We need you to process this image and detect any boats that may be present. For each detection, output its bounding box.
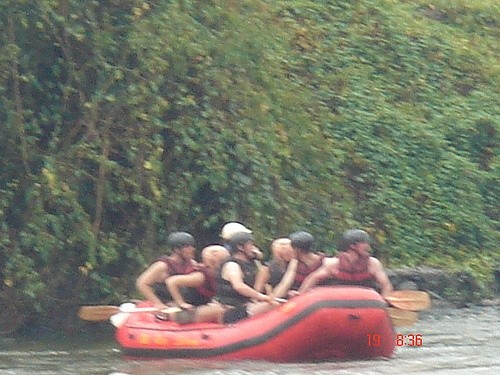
[114,284,397,363]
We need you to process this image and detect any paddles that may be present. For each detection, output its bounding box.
[78,305,182,321]
[272,296,417,326]
[383,289,431,311]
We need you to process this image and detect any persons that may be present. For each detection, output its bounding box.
[138,221,395,325]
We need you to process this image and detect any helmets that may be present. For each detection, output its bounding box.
[229,232,254,252]
[168,231,194,249]
[219,221,254,240]
[343,228,370,244]
[287,229,315,246]
